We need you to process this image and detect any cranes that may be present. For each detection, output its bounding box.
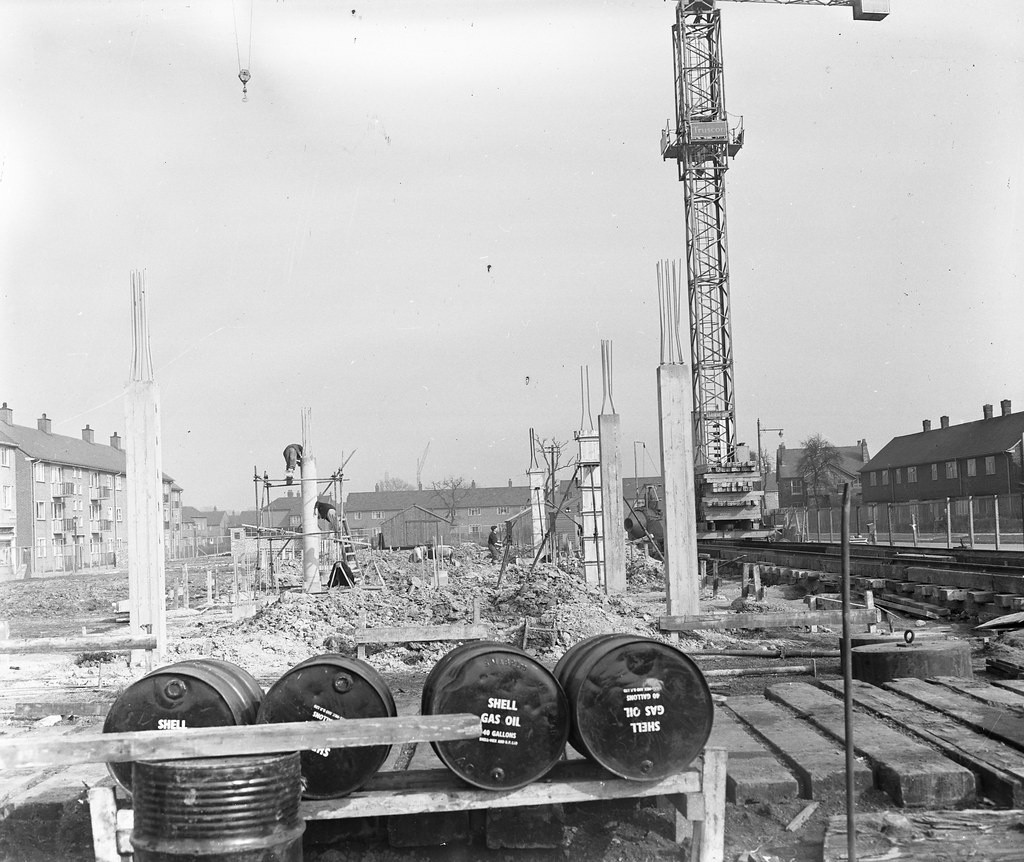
[658,1,889,540]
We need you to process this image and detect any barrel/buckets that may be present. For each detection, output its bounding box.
[421,641,571,791]
[103,658,264,796]
[128,752,306,862]
[553,633,715,783]
[255,654,398,800]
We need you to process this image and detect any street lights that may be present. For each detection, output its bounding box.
[757,418,784,527]
[634,440,646,507]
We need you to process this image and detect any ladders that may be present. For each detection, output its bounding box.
[335,515,363,577]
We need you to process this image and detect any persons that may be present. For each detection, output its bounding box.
[314,501,340,543]
[488,525,500,564]
[283,444,303,484]
